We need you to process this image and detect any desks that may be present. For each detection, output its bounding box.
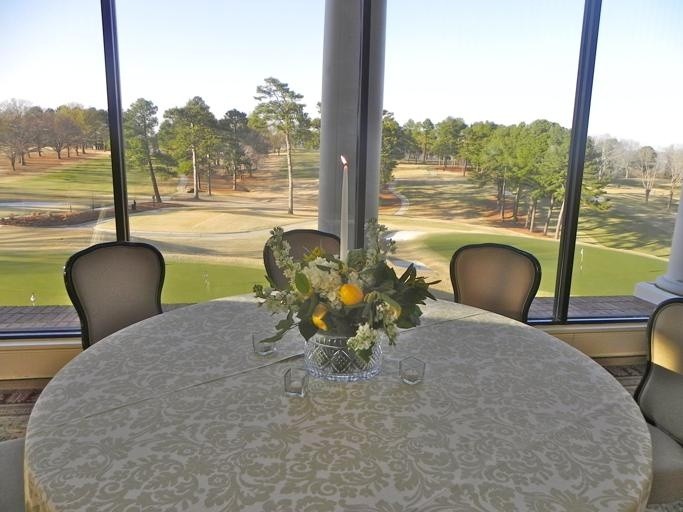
[23,289,655,512]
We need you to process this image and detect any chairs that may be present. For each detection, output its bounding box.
[449,242,542,324]
[62,240,166,352]
[634,296,682,503]
[262,230,340,288]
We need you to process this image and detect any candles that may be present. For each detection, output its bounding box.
[338,154,349,261]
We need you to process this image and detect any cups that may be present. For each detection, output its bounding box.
[253,332,275,354]
[400,359,425,385]
[285,367,309,397]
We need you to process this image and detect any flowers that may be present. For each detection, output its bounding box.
[250,218,442,377]
